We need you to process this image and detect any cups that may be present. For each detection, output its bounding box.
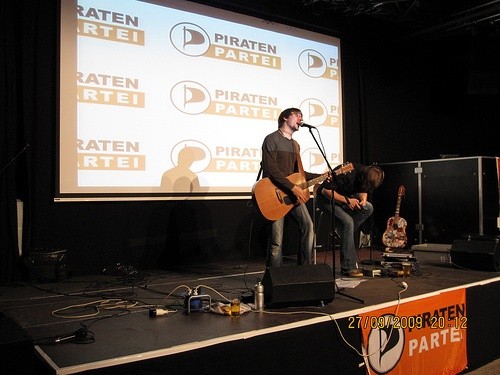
[402,262,411,278]
[231,299,241,319]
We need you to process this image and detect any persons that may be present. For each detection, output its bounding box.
[261,108,332,269]
[316,160,385,277]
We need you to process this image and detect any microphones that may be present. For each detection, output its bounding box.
[54,328,88,343]
[300,122,315,129]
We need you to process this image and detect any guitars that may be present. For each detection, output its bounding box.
[251,162,354,219]
[383,186,409,250]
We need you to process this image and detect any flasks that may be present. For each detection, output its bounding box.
[255,278,265,310]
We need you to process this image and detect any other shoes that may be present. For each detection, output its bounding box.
[330,227,341,240]
[344,270,364,277]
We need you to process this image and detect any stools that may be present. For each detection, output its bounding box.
[315,207,340,264]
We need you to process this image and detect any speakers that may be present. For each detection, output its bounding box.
[260,263,334,310]
[449,239,500,272]
